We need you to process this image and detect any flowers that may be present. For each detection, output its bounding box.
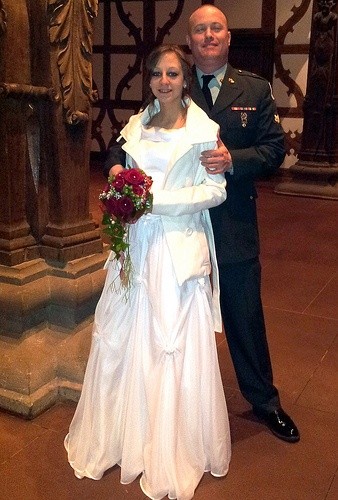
[99,167,155,303]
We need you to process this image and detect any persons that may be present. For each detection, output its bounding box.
[104,4,301,443]
[62,47,232,500]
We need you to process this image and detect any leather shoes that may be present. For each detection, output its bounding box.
[252,406,300,442]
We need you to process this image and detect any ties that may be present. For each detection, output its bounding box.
[201,75,215,111]
